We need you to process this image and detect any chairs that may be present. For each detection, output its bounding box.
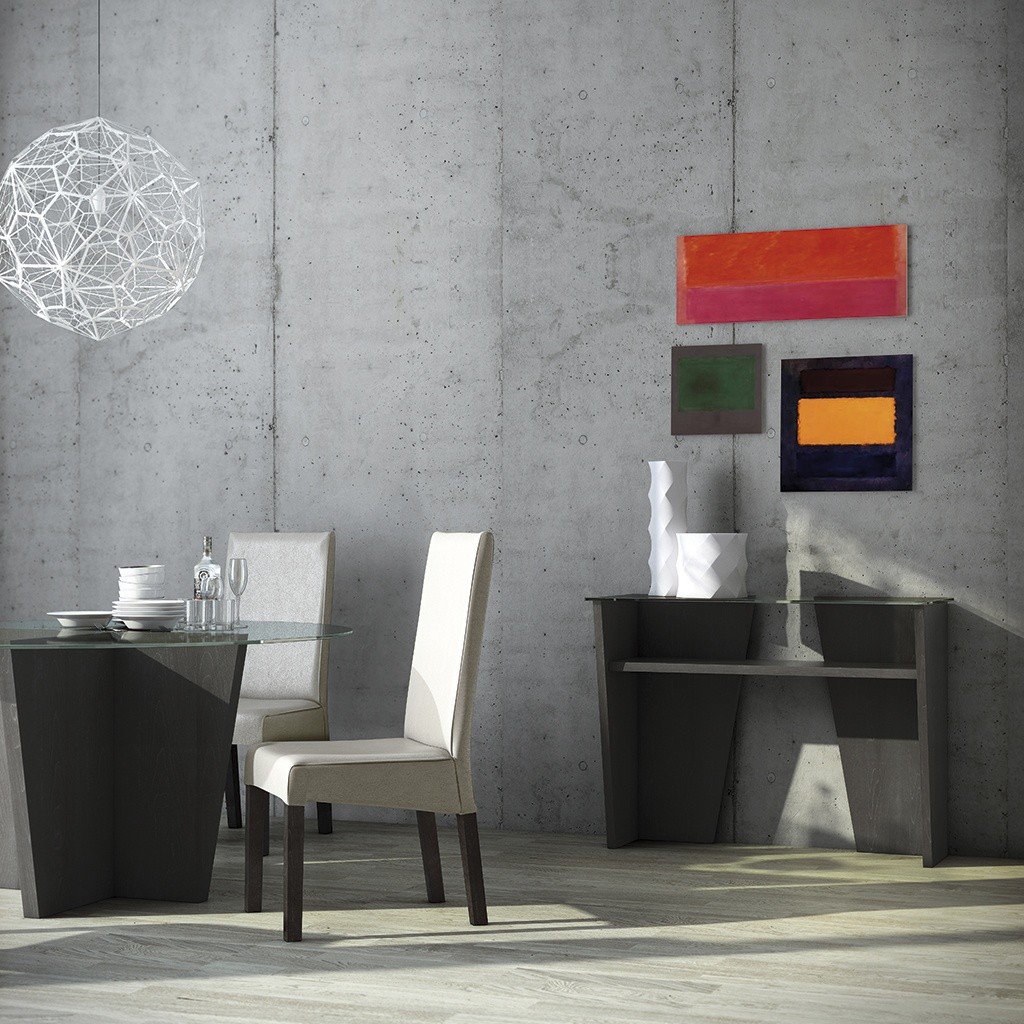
[244,528,496,942]
[216,529,334,834]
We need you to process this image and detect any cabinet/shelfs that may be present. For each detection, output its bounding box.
[587,592,954,869]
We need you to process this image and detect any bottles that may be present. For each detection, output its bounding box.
[192,536,221,600]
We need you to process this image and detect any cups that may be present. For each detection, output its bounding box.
[183,599,236,632]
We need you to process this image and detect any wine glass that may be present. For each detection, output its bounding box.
[228,558,249,628]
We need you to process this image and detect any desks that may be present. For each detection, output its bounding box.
[0,620,351,926]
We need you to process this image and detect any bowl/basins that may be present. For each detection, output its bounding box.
[46,611,112,629]
[112,599,186,632]
[118,564,166,600]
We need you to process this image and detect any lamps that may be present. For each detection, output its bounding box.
[0,0,207,341]
